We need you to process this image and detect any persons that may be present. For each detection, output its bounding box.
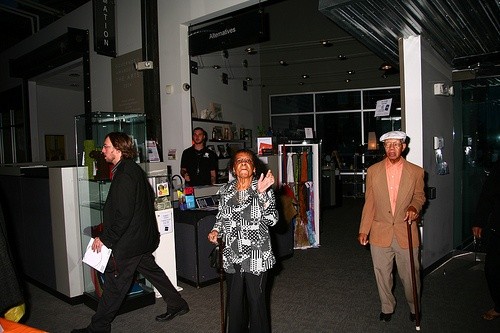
[180,127,219,186]
[71,132,190,333]
[358,131,427,323]
[207,149,280,333]
[471,142,500,322]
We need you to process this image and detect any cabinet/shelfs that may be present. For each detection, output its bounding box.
[192,116,248,184]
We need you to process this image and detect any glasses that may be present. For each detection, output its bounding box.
[102,145,114,149]
[383,142,402,148]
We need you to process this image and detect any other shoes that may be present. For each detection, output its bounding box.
[482,307,499,321]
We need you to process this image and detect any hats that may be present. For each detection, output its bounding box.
[379,131,406,142]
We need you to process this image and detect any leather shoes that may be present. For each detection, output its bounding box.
[379,312,391,322]
[409,311,422,322]
[71,323,94,333]
[156,306,190,321]
